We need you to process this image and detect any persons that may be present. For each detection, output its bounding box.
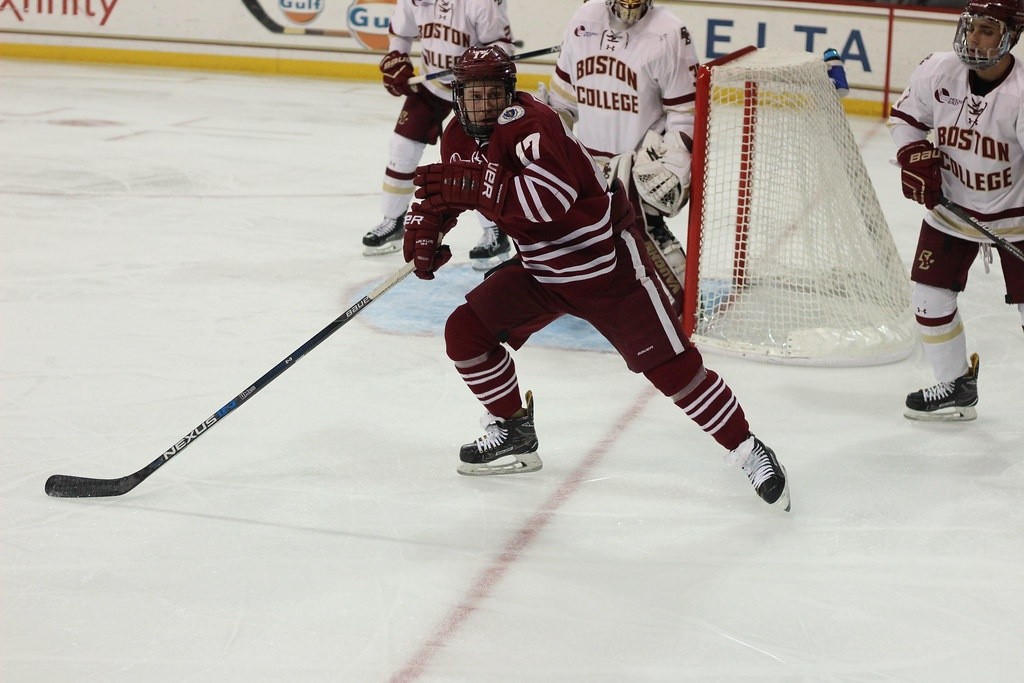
[888,0,1024,421]
[536,0,703,321]
[362,0,513,273]
[403,44,793,514]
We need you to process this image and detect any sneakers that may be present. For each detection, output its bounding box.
[728,431,791,512]
[904,352,980,422]
[469,225,514,270]
[458,390,544,475]
[363,213,408,256]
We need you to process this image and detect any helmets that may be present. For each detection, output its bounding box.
[454,45,517,140]
[965,0,1024,48]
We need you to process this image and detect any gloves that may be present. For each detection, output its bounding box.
[413,158,514,221]
[404,207,457,280]
[896,139,943,211]
[379,48,420,97]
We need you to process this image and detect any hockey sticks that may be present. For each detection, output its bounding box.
[936,194,1024,260]
[642,232,684,320]
[408,44,562,84]
[42,261,414,496]
